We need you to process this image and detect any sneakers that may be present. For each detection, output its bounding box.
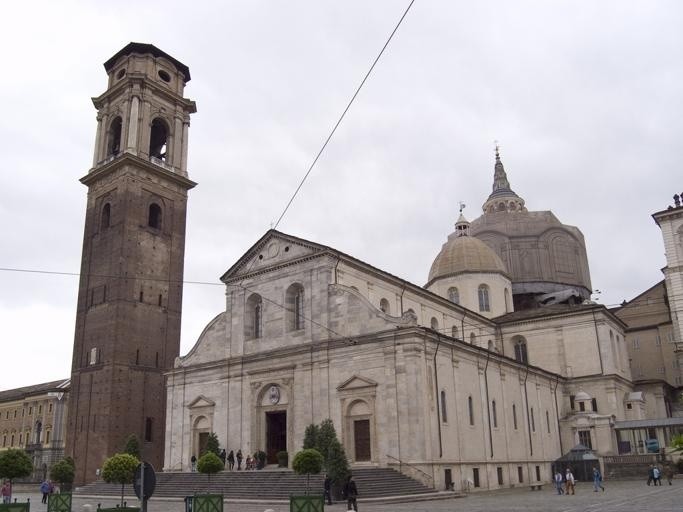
[593,488,604,492]
[565,492,574,495]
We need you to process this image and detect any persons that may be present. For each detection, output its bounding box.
[646,464,656,486]
[564,468,575,495]
[47,480,54,494]
[39,479,50,503]
[346,479,359,511]
[592,466,604,492]
[0,480,11,503]
[653,466,662,486]
[220,448,262,470]
[190,453,197,471]
[665,465,673,485]
[321,473,332,505]
[554,469,564,495]
